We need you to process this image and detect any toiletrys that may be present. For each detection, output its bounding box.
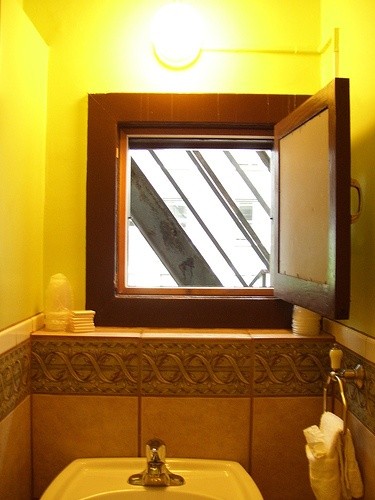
[44,273,74,332]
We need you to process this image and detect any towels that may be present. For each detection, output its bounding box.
[302,411,365,500]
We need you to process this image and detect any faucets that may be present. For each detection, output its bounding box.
[128,438,184,487]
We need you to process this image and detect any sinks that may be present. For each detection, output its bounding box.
[38,457,265,500]
